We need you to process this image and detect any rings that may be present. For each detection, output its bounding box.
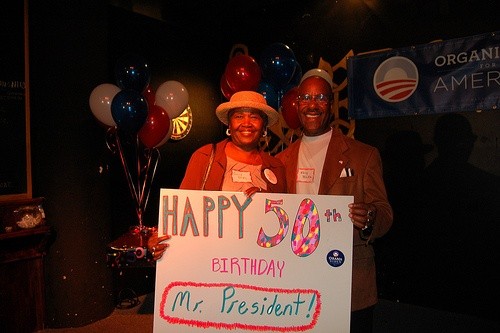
[368,210,373,217]
[152,251,155,256]
[151,246,156,251]
[362,225,368,230]
[367,219,371,224]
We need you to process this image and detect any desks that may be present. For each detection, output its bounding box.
[0,227,49,328]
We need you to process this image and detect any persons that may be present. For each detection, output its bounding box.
[274,75,393,333]
[148,91,285,262]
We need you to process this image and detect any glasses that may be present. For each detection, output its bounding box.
[296,93,330,104]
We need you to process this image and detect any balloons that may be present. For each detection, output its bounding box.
[89,60,189,149]
[220,44,300,111]
[281,87,302,130]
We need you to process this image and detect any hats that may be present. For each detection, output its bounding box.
[216,91,279,127]
